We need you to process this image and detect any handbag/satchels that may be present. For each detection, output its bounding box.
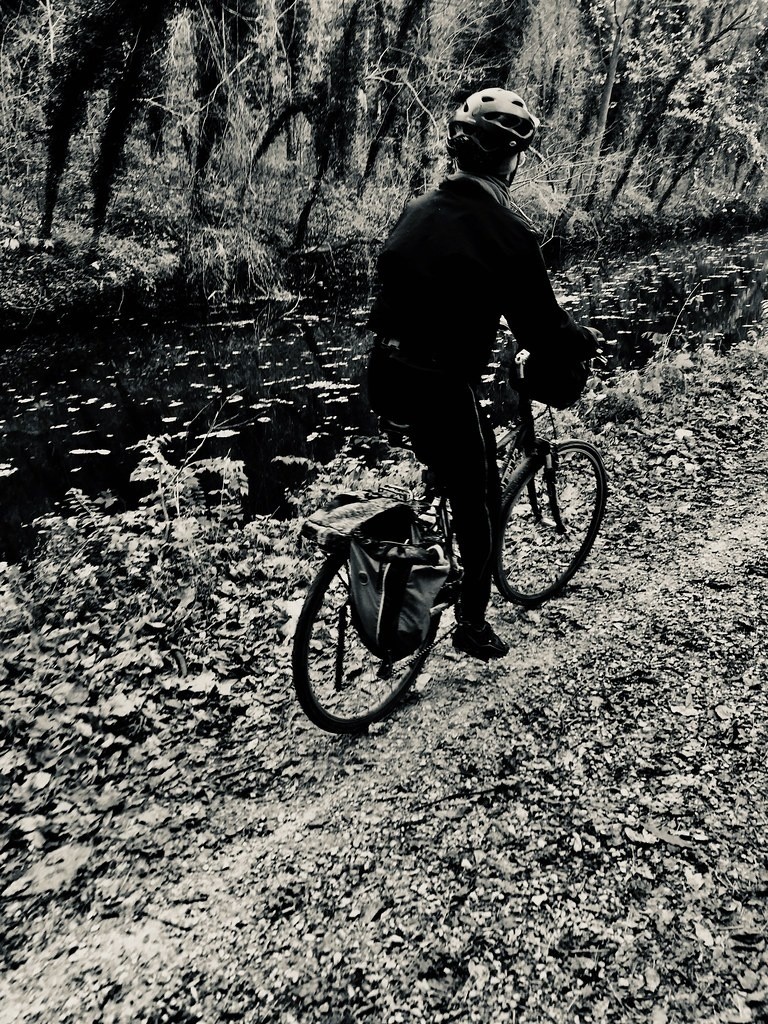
[299,490,449,664]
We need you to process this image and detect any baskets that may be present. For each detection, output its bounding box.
[492,340,590,410]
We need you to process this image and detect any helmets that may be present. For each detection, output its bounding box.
[444,89,534,160]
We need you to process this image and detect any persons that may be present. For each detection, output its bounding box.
[371,87,603,658]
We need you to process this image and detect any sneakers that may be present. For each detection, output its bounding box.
[453,620,511,661]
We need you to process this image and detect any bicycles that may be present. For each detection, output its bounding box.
[290,330,623,733]
